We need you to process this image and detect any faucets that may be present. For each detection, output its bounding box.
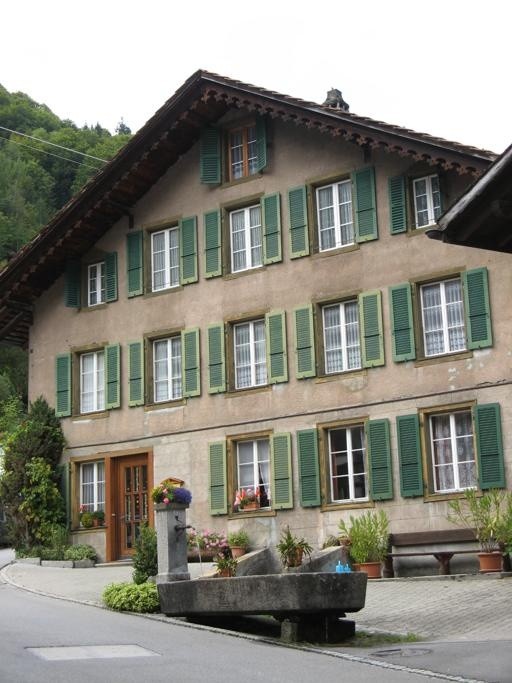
[175,525,196,530]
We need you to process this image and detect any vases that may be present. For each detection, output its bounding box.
[241,501,258,512]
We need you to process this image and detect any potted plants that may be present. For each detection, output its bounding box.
[228,532,249,558]
[79,512,105,528]
[449,486,509,573]
[276,525,310,568]
[213,556,242,577]
[344,508,385,579]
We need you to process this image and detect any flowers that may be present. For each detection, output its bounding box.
[152,484,194,505]
[234,487,258,501]
[186,529,228,548]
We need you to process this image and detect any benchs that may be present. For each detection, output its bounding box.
[386,524,512,578]
